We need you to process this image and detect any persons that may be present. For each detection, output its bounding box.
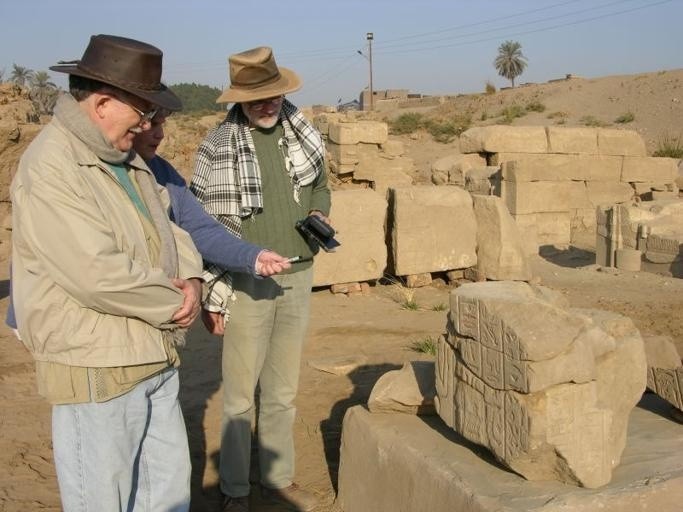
[7,105,292,340]
[9,36,203,512]
[189,46,330,512]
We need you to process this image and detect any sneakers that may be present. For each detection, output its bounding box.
[257,484,319,511]
[221,495,248,512]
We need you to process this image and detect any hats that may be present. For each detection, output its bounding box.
[215,47,301,104]
[49,34,182,110]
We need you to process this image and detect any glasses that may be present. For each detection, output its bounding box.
[246,95,285,111]
[102,91,158,122]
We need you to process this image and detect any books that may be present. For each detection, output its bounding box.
[295,220,341,253]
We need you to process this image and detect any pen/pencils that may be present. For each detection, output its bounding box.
[275,255,302,265]
